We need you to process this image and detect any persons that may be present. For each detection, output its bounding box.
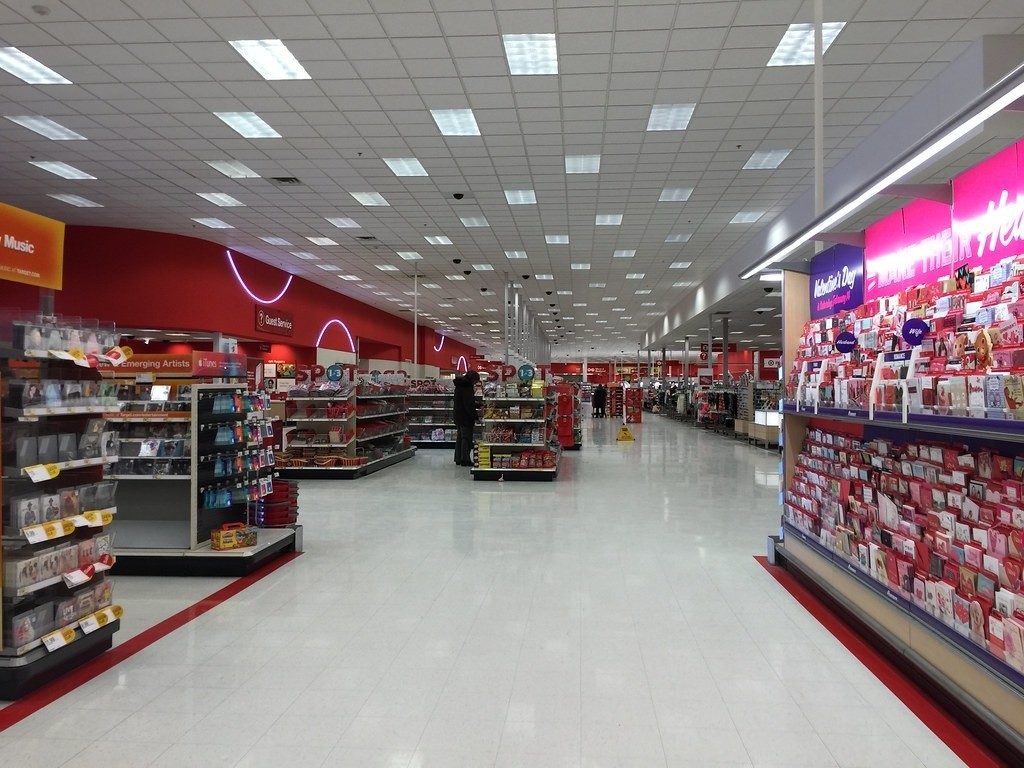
[44,555,58,575]
[671,380,676,390]
[594,384,607,418]
[20,561,37,581]
[106,433,114,456]
[27,384,41,405]
[23,616,35,643]
[24,502,36,526]
[452,370,481,467]
[46,498,57,521]
[938,337,947,356]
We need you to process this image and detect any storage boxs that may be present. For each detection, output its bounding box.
[211,523,259,552]
[473,444,512,469]
[249,476,300,529]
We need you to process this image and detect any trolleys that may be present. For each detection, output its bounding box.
[591,395,607,419]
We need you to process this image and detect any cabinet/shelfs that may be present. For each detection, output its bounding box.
[470,383,562,482]
[542,381,583,451]
[606,388,624,419]
[0,295,121,700]
[738,62,1024,758]
[407,387,458,448]
[107,384,304,576]
[272,384,417,480]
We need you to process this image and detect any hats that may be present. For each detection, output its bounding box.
[48,498,54,502]
[27,502,31,507]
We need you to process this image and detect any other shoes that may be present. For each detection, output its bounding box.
[456,462,460,465]
[460,461,473,466]
